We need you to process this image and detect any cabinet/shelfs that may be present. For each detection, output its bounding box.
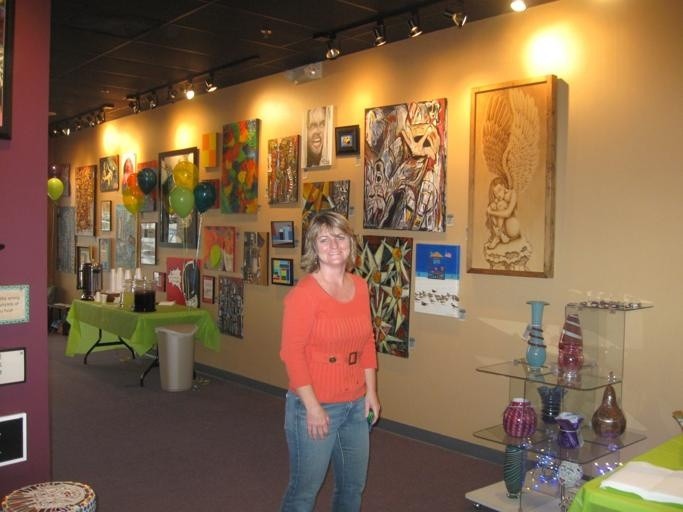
[465,363,646,512]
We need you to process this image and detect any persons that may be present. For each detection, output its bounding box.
[280,212,380,512]
[485,177,521,248]
[306,106,326,167]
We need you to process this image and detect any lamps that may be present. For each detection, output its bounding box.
[49,1,469,137]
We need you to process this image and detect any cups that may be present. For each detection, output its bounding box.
[93,291,101,303]
[100,294,109,304]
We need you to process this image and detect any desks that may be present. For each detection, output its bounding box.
[65,299,221,390]
[570,434,683,512]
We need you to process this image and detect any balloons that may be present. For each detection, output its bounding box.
[47,178,64,201]
[123,161,216,218]
[48,163,64,177]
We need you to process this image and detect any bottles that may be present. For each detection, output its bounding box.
[132,277,159,313]
[120,279,132,313]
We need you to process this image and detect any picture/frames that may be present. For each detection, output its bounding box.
[0,285,30,325]
[270,219,294,248]
[75,245,93,278]
[0,0,14,140]
[201,275,215,304]
[335,126,360,156]
[0,348,27,385]
[271,258,293,286]
[0,412,29,466]
[465,74,558,277]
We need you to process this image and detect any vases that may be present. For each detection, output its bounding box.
[503,301,626,498]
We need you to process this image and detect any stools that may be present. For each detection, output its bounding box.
[0,481,98,512]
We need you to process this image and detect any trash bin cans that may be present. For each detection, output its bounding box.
[154,325,198,393]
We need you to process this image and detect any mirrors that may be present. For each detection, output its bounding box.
[158,147,198,251]
